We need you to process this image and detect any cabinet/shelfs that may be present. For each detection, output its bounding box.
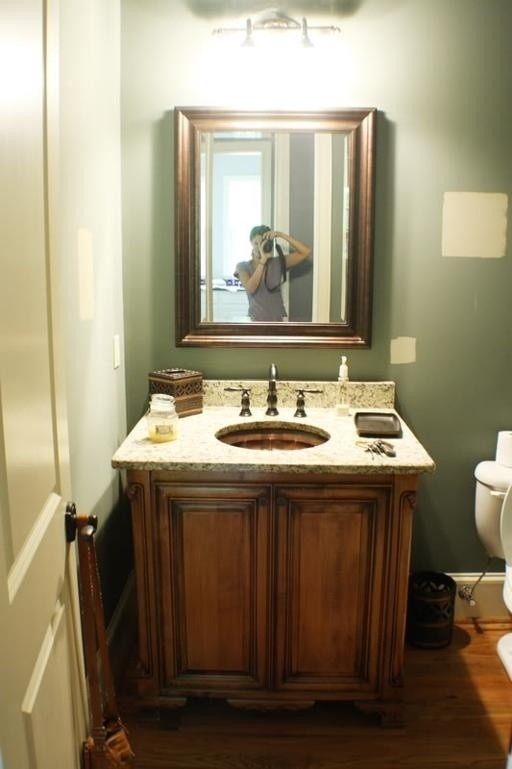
[126,467,396,703]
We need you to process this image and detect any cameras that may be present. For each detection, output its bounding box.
[260,238,273,254]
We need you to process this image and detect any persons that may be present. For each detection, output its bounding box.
[232,226,312,323]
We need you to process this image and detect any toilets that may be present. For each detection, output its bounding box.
[473,459,512,683]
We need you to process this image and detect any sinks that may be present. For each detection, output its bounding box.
[217,421,331,450]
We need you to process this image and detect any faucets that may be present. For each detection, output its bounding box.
[265,364,279,416]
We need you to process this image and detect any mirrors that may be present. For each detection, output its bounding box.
[170,103,378,350]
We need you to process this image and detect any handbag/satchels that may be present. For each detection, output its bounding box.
[83,715,135,769]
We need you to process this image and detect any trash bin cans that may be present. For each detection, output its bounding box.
[408,571,456,648]
